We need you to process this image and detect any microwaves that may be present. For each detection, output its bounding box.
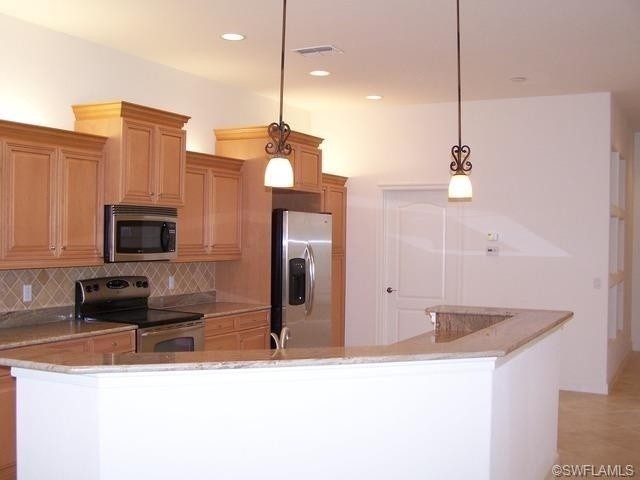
[104,204,178,263]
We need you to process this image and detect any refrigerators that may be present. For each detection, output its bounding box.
[272,209,332,348]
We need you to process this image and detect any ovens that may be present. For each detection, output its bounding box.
[134,319,206,353]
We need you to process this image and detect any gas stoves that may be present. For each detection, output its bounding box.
[81,307,204,327]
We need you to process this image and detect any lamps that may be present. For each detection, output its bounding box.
[447,0,473,203]
[264,2,294,188]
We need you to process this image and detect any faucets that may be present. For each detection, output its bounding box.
[271,349,288,360]
[270,327,292,349]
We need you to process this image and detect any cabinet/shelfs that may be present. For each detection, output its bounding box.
[70,100,193,208]
[208,309,270,351]
[322,173,350,347]
[213,127,326,193]
[0,330,135,480]
[0,120,105,270]
[176,150,251,261]
